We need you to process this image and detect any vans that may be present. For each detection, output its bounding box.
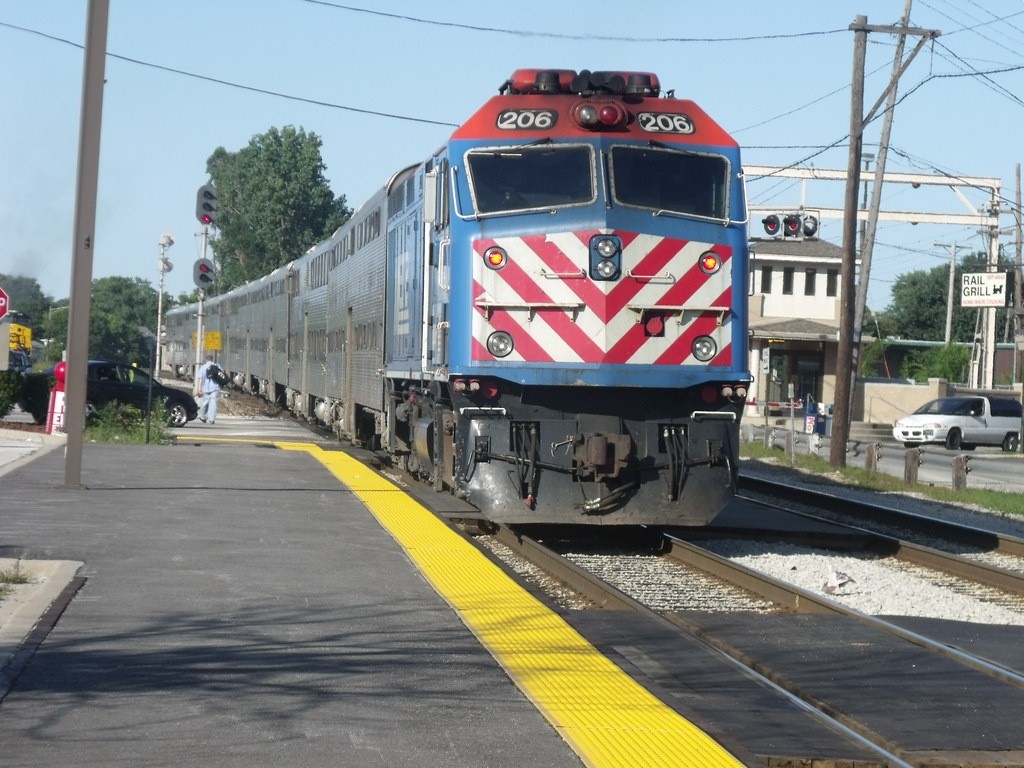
[893,398,1023,452]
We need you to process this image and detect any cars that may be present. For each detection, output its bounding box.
[18,360,198,427]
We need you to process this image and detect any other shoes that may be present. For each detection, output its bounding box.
[198,417,207,423]
[210,420,215,423]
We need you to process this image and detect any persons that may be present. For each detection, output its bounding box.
[198,355,223,423]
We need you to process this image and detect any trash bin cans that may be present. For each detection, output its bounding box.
[826,404,835,437]
[804,402,827,434]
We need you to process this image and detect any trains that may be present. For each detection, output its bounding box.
[165,69,753,525]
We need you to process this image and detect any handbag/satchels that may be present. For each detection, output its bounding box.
[210,364,230,386]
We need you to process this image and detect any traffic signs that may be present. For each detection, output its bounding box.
[762,347,770,374]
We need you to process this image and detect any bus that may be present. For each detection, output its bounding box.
[8,309,32,355]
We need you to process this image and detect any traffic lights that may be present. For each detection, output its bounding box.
[195,185,218,224]
[192,258,214,288]
[762,214,780,235]
[784,215,802,235]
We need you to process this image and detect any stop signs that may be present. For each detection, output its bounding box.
[0,287,10,321]
